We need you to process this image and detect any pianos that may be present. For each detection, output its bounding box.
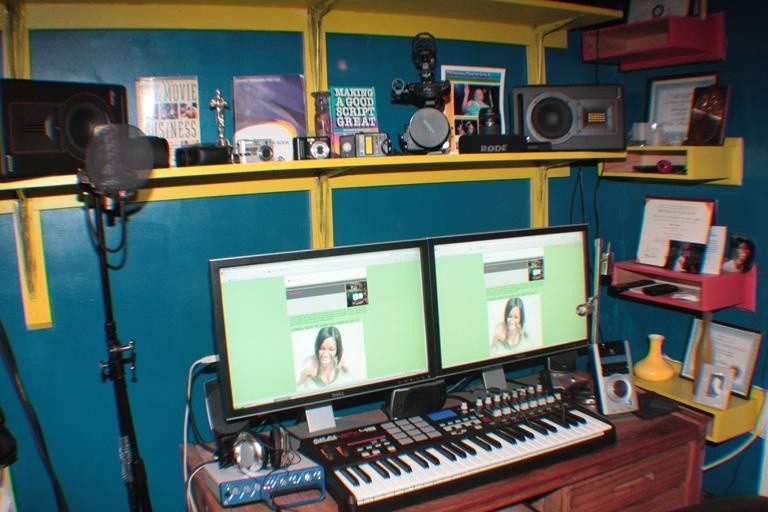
[299,381,618,511]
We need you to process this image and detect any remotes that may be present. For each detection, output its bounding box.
[615,279,656,290]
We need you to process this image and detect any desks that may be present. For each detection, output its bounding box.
[182,369,715,512]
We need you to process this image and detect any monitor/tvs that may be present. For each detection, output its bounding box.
[426,221,593,406]
[209,239,433,448]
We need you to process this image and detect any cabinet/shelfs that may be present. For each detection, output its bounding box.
[597,135,745,186]
[632,349,768,444]
[582,11,728,73]
[608,258,759,313]
[0,0,624,332]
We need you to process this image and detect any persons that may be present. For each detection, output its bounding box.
[461,83,489,117]
[298,327,351,388]
[709,376,722,398]
[720,238,755,274]
[489,297,530,351]
[454,121,475,136]
[672,248,694,273]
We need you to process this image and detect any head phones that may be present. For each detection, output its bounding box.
[212,420,301,476]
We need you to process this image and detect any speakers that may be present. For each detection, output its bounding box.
[0,77,129,182]
[390,379,447,419]
[592,339,640,416]
[509,84,627,151]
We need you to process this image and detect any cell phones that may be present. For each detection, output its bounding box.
[642,284,678,295]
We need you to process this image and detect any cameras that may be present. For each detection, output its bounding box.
[340,136,355,158]
[459,108,527,153]
[293,136,331,160]
[356,133,391,157]
[238,139,276,163]
[391,32,451,154]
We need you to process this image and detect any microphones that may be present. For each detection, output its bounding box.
[70,125,154,267]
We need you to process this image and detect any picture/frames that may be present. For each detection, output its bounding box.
[678,317,763,400]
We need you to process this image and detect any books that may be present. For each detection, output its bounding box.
[232,73,311,161]
[134,75,201,168]
[328,84,379,158]
